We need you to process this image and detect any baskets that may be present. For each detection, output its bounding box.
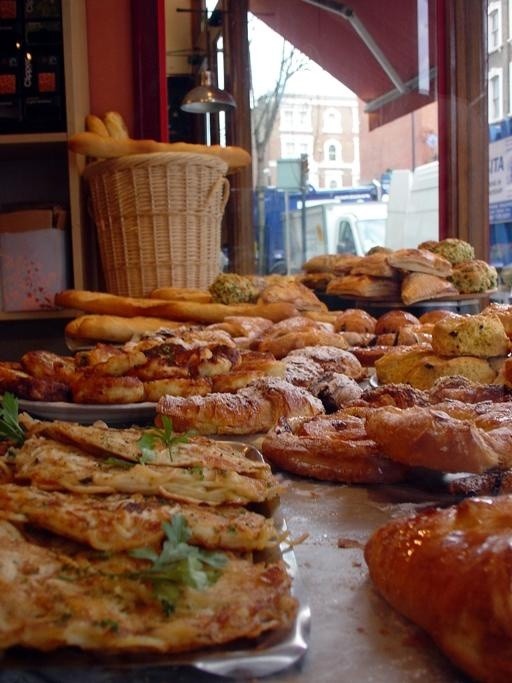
[86,150,230,297]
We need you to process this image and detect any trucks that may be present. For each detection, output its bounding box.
[280,200,386,274]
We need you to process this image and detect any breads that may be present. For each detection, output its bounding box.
[68,111,252,168]
[0,237,512,683]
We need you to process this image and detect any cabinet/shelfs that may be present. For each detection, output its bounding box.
[0,0,92,321]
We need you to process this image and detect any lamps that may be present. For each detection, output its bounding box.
[180,8,237,115]
